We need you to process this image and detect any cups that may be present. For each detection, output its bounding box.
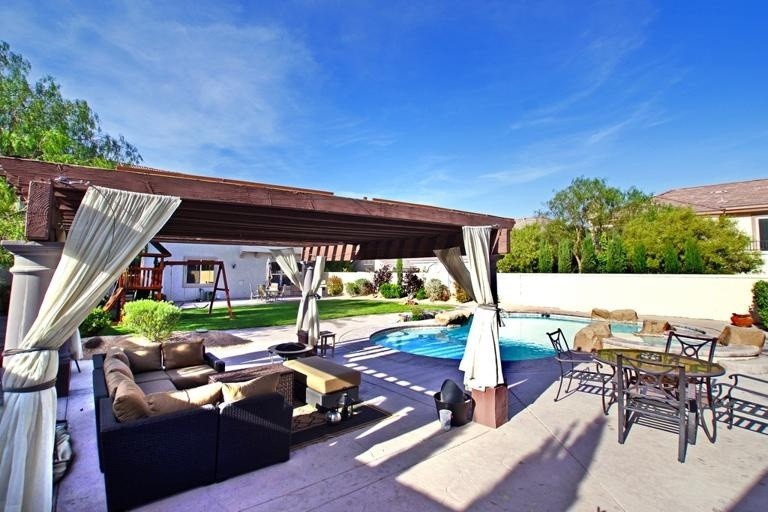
[438,409,453,433]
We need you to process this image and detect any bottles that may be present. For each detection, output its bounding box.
[338,393,354,421]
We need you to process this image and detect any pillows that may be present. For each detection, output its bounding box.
[161,338,205,370]
[220,369,281,403]
[103,357,135,383]
[112,378,148,423]
[104,346,130,368]
[143,381,222,416]
[123,344,161,376]
[104,371,131,398]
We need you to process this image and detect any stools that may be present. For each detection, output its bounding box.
[316,331,336,358]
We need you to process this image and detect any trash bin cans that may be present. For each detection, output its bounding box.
[434,377,476,430]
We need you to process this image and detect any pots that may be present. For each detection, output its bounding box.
[324,408,342,425]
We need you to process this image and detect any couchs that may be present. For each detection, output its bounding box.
[90,345,292,512]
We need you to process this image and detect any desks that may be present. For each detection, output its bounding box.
[587,347,724,407]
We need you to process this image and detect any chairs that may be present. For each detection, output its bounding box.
[710,370,768,447]
[545,327,614,415]
[615,351,695,464]
[664,330,720,412]
[249,282,286,303]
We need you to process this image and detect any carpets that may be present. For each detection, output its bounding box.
[288,397,392,452]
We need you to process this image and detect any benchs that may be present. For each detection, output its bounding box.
[282,356,363,425]
